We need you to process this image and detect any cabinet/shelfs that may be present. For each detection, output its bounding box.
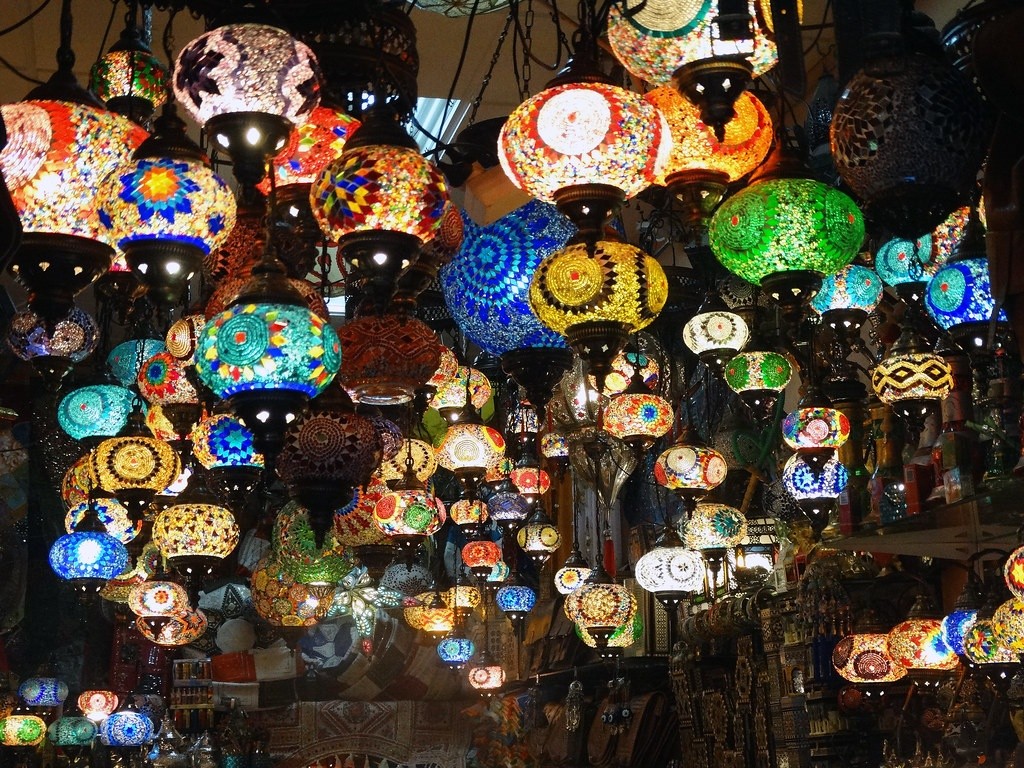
[169,659,214,733]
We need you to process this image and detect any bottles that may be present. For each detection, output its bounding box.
[175,662,210,679]
[170,688,207,704]
[174,709,214,730]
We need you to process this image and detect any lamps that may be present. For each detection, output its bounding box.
[1,0,1021,768]
